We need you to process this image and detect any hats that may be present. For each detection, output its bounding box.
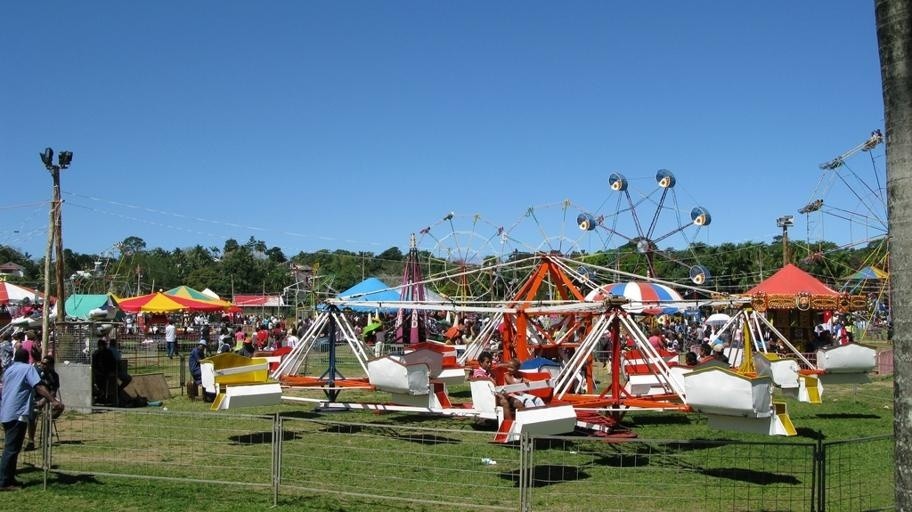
[243,339,252,344]
[197,340,208,347]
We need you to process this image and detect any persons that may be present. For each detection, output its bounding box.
[66,313,165,336]
[0,347,66,493]
[166,312,314,359]
[91,339,131,402]
[0,296,60,419]
[640,308,731,365]
[335,308,498,345]
[474,352,545,420]
[766,293,892,365]
[189,340,206,395]
[528,319,611,394]
[23,354,65,451]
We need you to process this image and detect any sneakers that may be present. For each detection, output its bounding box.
[0,480,24,492]
[23,442,35,451]
